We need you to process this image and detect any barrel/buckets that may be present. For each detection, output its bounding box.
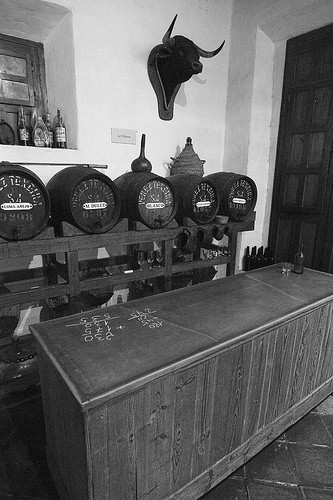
[166,174,220,224]
[0,162,51,241]
[46,167,121,234]
[206,172,257,222]
[113,171,179,230]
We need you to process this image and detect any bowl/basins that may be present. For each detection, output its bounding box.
[215,216,229,224]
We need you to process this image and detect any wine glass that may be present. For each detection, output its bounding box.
[138,252,144,272]
[148,251,155,269]
[156,251,164,268]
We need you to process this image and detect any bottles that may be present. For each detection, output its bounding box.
[243,246,272,271]
[55,109,67,148]
[46,114,53,147]
[30,108,47,146]
[295,243,305,272]
[131,134,152,172]
[18,108,30,146]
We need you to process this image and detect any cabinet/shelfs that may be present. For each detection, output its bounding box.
[0,210,257,415]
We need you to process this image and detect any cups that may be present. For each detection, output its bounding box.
[224,225,233,237]
[195,227,213,245]
[212,226,223,240]
[174,228,195,255]
[200,249,209,261]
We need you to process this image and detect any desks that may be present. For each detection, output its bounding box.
[27,260,333,500]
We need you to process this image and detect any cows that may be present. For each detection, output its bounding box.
[156,13,226,110]
